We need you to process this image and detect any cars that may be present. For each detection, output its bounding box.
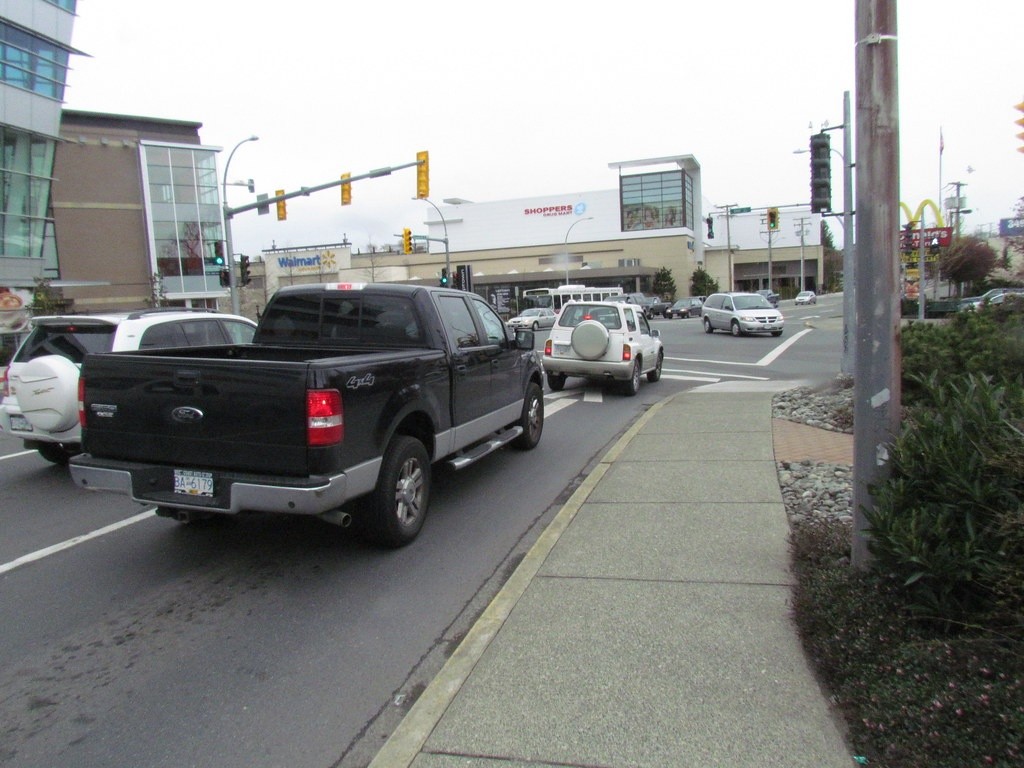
[506,307,560,332]
[755,289,780,308]
[665,297,703,319]
[794,290,816,306]
[900,288,1024,320]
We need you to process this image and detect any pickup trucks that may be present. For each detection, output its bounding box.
[66,282,546,549]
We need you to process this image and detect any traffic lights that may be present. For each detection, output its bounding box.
[403,227,413,255]
[706,217,715,240]
[930,237,940,255]
[900,223,914,256]
[766,208,779,230]
[439,266,449,287]
[218,270,231,287]
[240,254,252,285]
[214,240,225,266]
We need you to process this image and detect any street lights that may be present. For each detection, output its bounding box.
[565,216,594,285]
[412,196,451,287]
[220,136,261,343]
[946,208,973,298]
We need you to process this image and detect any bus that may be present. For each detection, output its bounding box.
[522,285,623,325]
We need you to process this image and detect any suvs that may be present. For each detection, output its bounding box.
[588,291,674,324]
[541,299,665,397]
[0,304,259,464]
[701,291,785,337]
